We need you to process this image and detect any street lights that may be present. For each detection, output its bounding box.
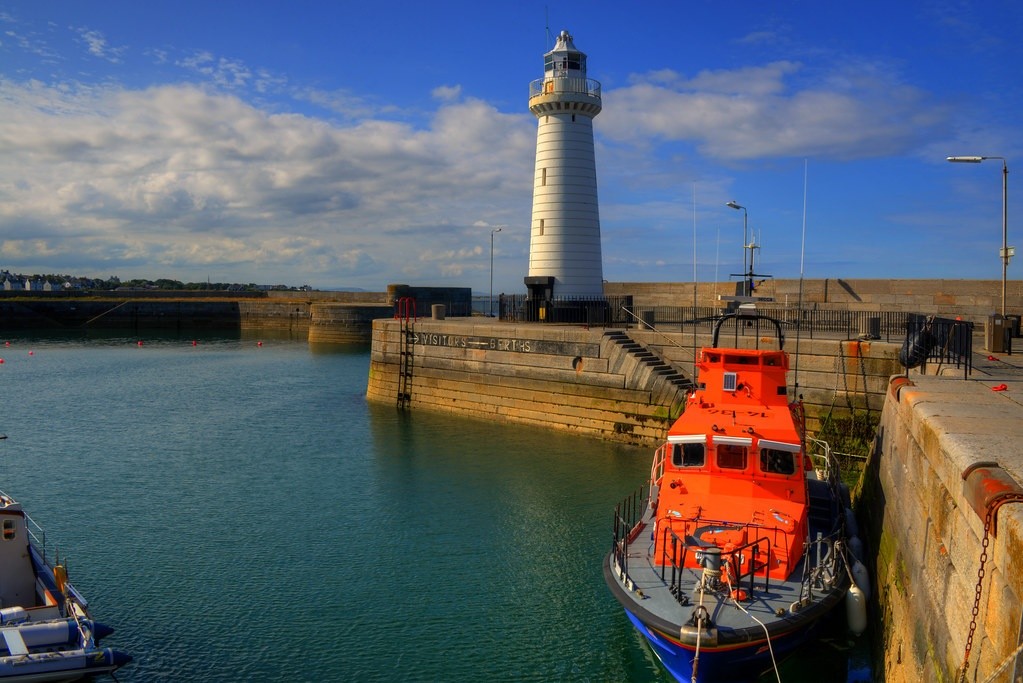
[487,227,501,318]
[726,201,747,296]
[947,154,1008,320]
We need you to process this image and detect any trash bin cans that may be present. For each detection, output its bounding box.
[984,313,1004,352]
[1006,314,1021,338]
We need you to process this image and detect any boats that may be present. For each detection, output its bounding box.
[603,315,869,682]
[0,481,116,683]
